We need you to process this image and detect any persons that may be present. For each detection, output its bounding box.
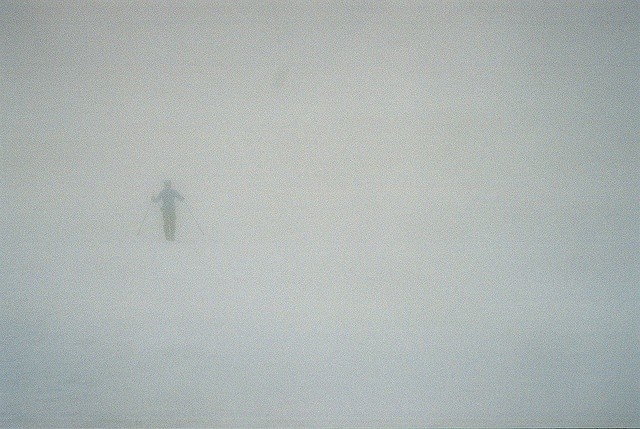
[151,178,184,242]
